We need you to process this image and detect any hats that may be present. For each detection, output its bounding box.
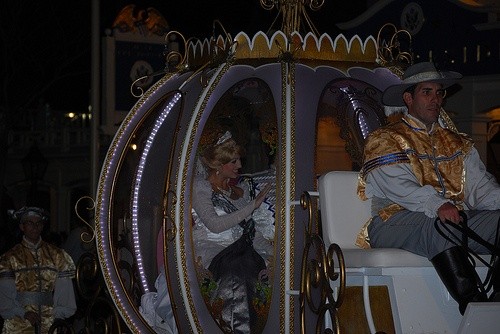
[14,206,48,222]
[382,62,463,107]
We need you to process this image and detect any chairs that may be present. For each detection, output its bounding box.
[300,168,500,334]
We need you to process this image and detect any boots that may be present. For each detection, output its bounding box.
[431,247,489,317]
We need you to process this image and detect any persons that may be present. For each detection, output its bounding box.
[357,62,500,318]
[0,205,78,334]
[139,122,276,334]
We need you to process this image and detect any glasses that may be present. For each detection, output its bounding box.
[22,220,42,225]
